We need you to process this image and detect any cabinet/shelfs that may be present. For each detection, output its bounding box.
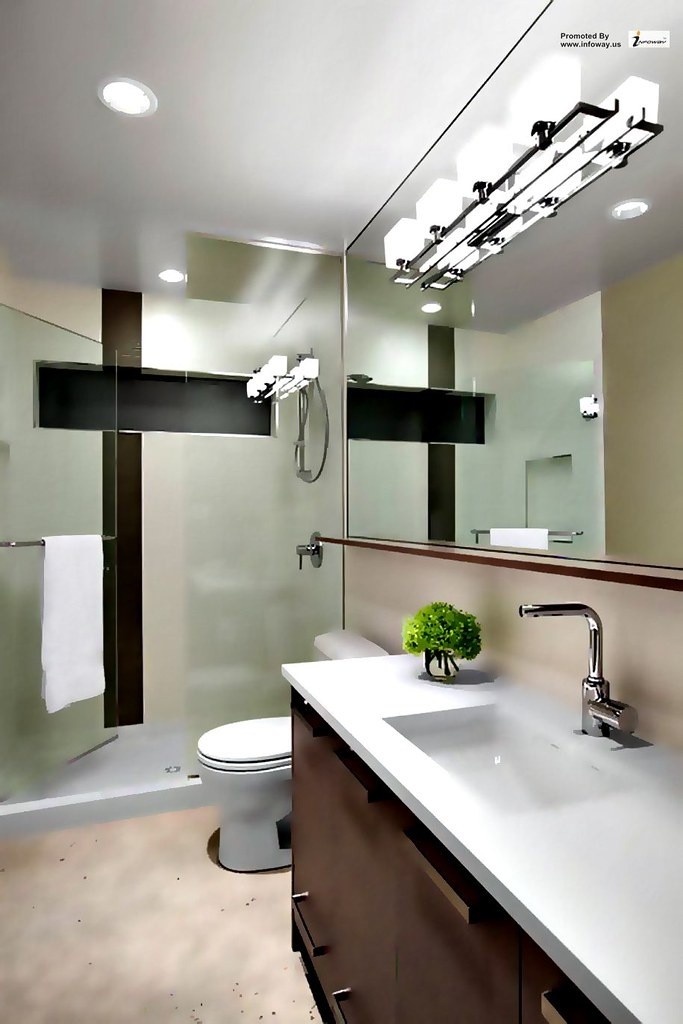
[291,685,610,1024]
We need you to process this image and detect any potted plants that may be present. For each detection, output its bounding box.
[401,602,483,682]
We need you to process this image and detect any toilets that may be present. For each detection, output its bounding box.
[196,628,390,873]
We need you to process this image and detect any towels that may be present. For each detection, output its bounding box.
[489,528,549,551]
[40,534,105,714]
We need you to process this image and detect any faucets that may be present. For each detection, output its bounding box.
[518,602,640,739]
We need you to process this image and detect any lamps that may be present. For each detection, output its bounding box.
[383,54,664,293]
[246,354,319,403]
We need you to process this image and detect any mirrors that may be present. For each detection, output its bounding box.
[346,0,683,570]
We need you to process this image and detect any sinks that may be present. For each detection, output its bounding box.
[380,702,646,818]
